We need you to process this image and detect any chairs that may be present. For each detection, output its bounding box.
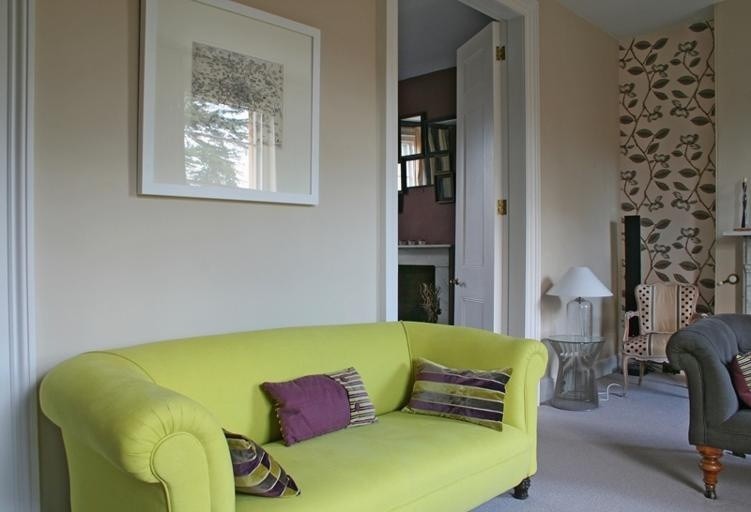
[621,280,709,398]
[664,312,751,500]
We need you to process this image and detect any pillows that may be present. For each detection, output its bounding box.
[726,349,751,410]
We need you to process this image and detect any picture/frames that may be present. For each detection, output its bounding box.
[137,0,322,210]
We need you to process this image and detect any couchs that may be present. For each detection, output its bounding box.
[35,319,552,512]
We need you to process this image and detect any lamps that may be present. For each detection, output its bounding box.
[544,265,614,338]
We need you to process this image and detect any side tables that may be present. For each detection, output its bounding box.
[545,334,605,412]
